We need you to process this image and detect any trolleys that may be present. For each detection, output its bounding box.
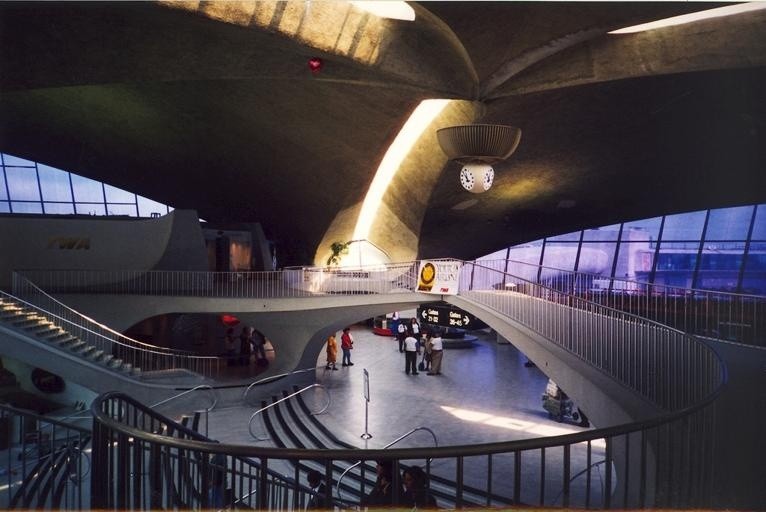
[548,391,579,422]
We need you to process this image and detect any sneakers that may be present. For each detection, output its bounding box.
[326,366,332,369]
[342,362,354,366]
[333,367,338,370]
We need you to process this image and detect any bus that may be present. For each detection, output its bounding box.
[635,248,766,289]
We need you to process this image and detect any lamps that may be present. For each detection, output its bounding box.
[433,100,523,194]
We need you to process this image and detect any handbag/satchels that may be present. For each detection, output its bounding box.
[418,361,424,371]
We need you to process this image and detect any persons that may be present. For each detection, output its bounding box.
[364,458,404,511]
[206,439,226,510]
[340,328,354,366]
[397,317,443,376]
[305,470,327,512]
[326,332,339,370]
[250,330,267,364]
[396,465,438,512]
[225,328,240,367]
[239,326,252,367]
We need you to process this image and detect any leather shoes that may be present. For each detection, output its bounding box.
[427,372,441,375]
